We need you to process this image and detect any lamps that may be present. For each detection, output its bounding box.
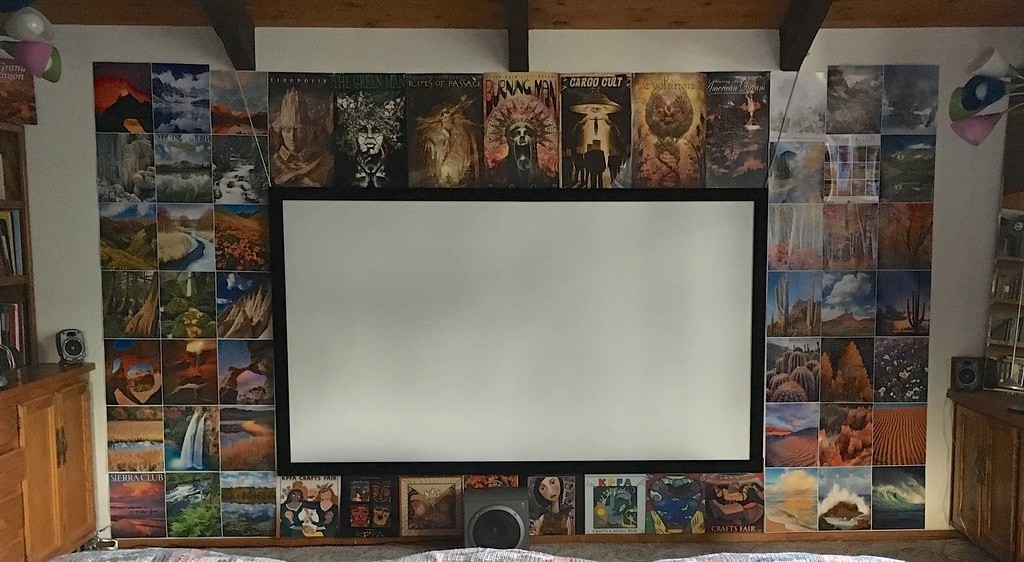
[1,6,64,85]
[950,45,1023,147]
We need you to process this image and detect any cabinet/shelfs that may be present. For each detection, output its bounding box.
[1,123,39,373]
[2,360,99,562]
[947,385,1024,562]
[979,68,1023,395]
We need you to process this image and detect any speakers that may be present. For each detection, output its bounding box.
[55,329,86,367]
[462,488,530,550]
[951,356,998,391]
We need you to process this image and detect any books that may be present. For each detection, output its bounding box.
[989,308,1024,344]
[0,146,24,202]
[986,350,1024,391]
[1,208,28,279]
[0,301,29,369]
[994,263,1024,304]
[998,219,1023,259]
